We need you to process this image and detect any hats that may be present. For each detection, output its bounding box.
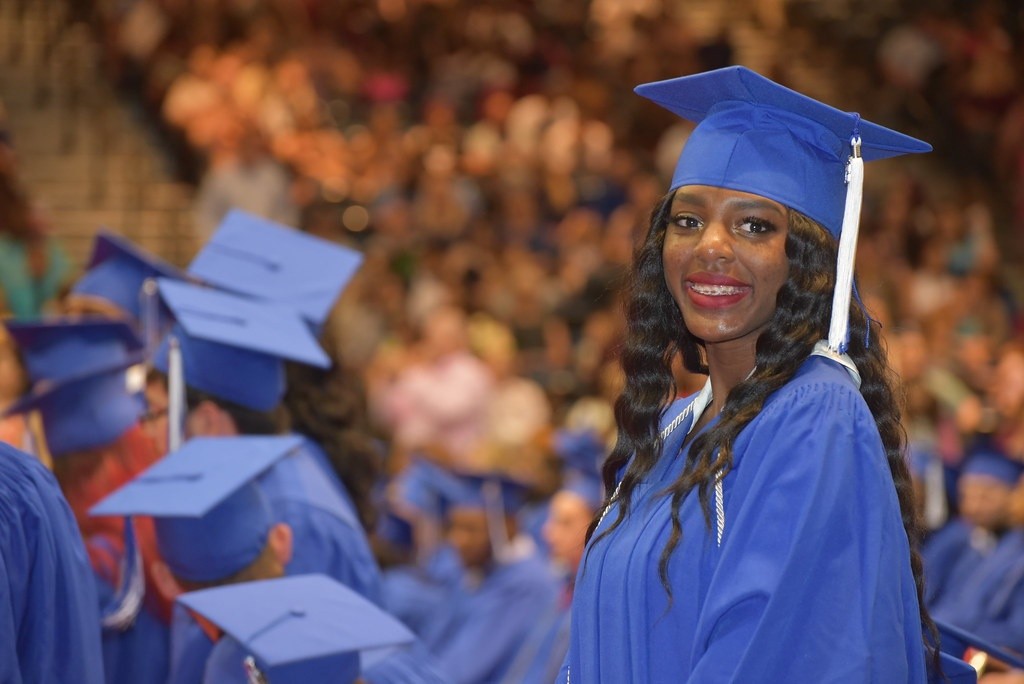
[2,315,147,456]
[634,64,933,356]
[88,433,416,684]
[958,444,1020,484]
[71,208,365,418]
[368,428,630,550]
[925,612,1024,684]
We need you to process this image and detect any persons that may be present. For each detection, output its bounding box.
[898,430,1024,684]
[364,431,614,684]
[548,64,924,684]
[0,0,1024,484]
[0,206,426,684]
